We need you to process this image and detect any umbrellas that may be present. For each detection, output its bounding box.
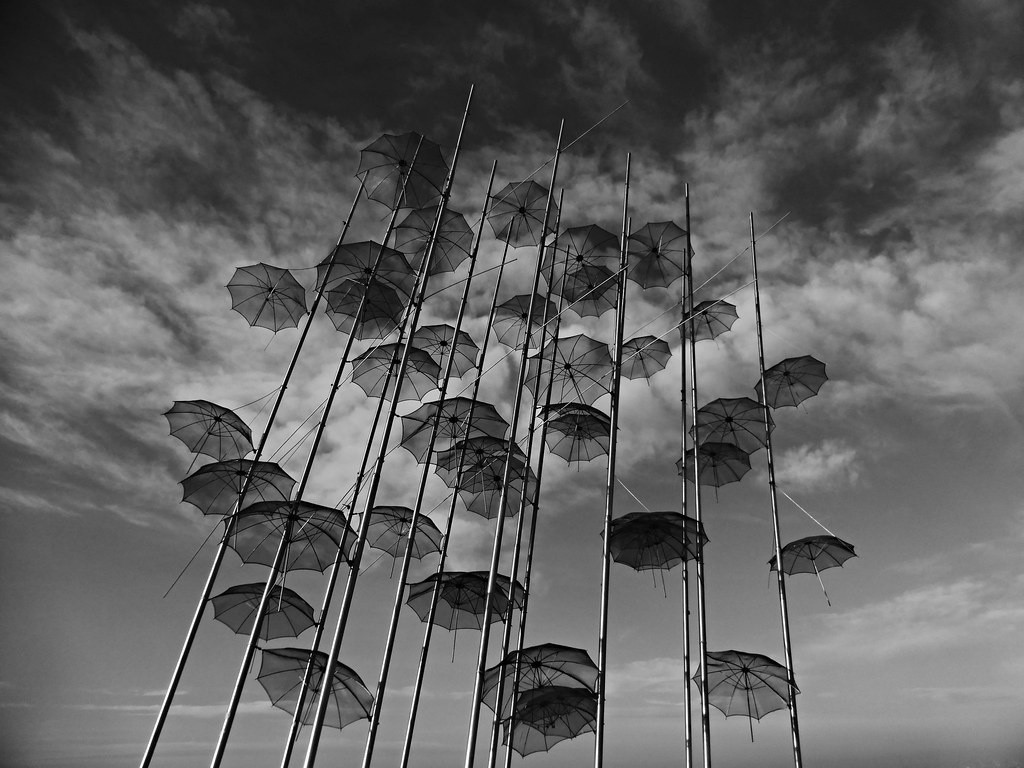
[258,648,374,743]
[690,649,801,742]
[477,642,599,722]
[766,534,860,608]
[754,352,829,415]
[497,683,598,758]
[155,131,777,641]
